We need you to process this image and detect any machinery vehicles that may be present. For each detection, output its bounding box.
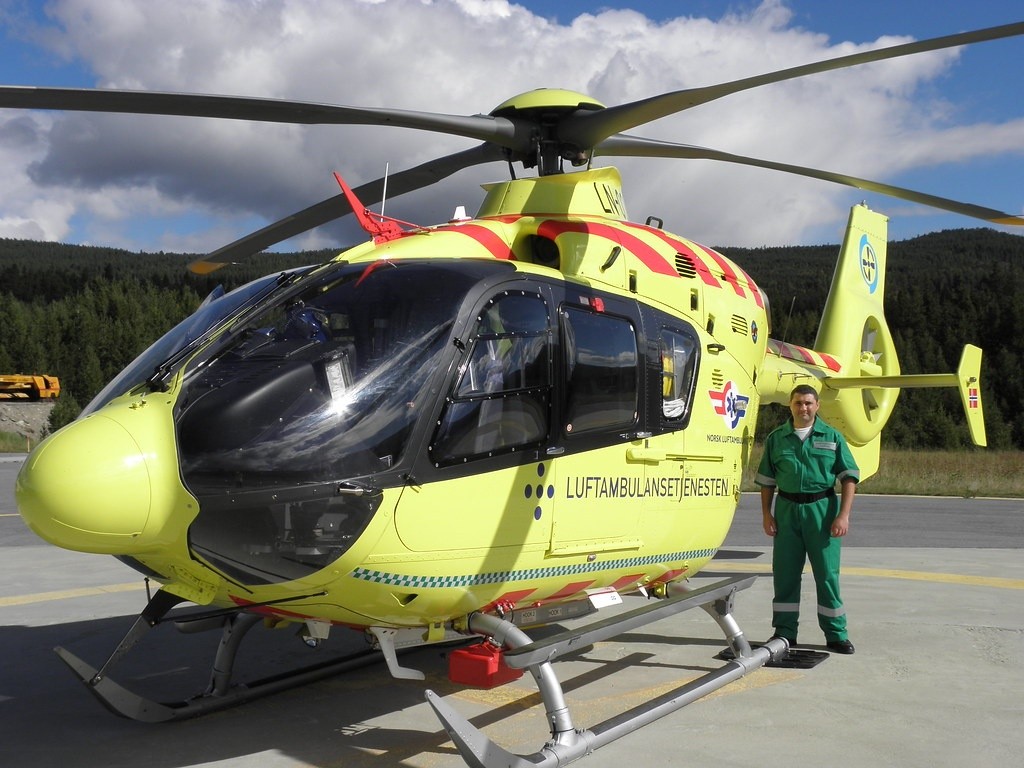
[1,374,60,402]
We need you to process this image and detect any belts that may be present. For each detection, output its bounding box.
[779,488,834,503]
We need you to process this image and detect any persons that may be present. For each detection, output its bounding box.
[754,385,860,654]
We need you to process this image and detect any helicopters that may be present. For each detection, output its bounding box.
[1,21,1020,768]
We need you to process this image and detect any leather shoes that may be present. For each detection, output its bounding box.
[768,635,798,646]
[826,640,855,654]
